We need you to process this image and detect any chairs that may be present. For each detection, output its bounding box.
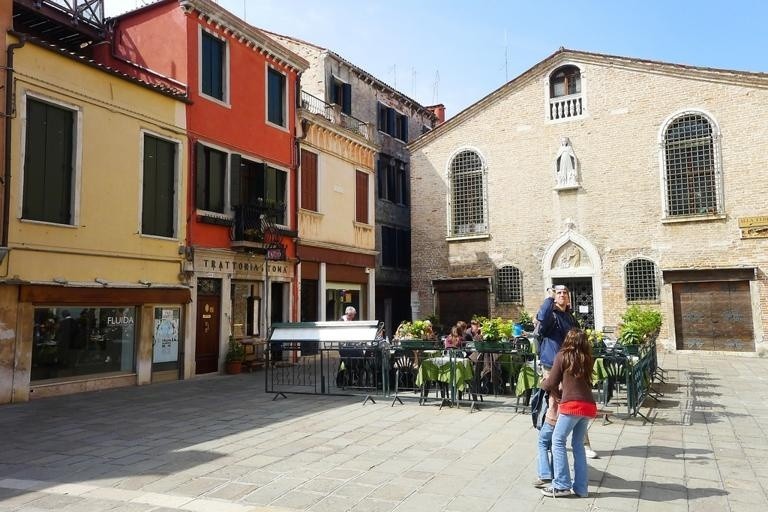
[337,332,647,409]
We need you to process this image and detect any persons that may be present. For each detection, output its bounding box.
[554,138,579,186]
[34,309,124,372]
[535,285,597,459]
[570,244,581,267]
[540,329,597,497]
[337,307,357,354]
[533,375,561,489]
[560,250,569,265]
[375,317,482,361]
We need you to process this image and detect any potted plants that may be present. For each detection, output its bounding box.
[397,321,439,348]
[471,313,515,351]
[616,321,641,354]
[224,334,244,374]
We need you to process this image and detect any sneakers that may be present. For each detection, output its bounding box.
[584,445,597,458]
[532,479,588,498]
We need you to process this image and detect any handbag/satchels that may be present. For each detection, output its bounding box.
[530,389,550,430]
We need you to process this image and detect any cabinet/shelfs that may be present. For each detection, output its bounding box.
[241,339,268,371]
